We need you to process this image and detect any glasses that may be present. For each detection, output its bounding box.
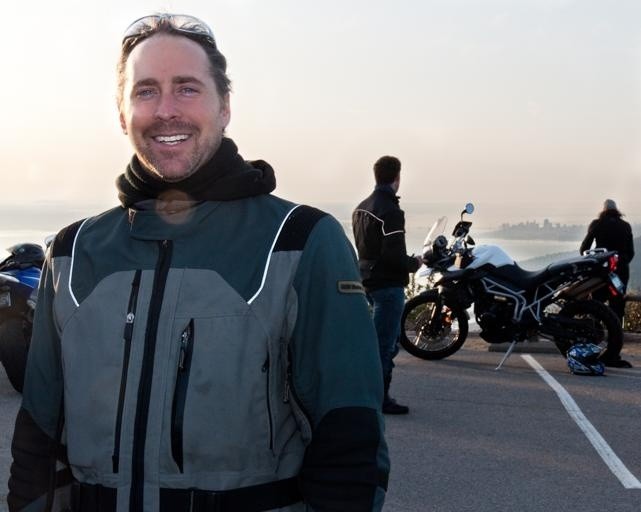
[120,13,217,51]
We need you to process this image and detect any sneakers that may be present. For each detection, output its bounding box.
[606,356,633,367]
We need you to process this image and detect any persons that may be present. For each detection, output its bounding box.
[352,155,423,414]
[579,199,635,367]
[6,14,390,512]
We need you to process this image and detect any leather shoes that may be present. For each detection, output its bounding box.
[387,405,410,416]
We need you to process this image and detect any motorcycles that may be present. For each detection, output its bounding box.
[397,203,624,366]
[0,233,55,395]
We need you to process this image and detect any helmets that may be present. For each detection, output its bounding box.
[566,343,608,375]
